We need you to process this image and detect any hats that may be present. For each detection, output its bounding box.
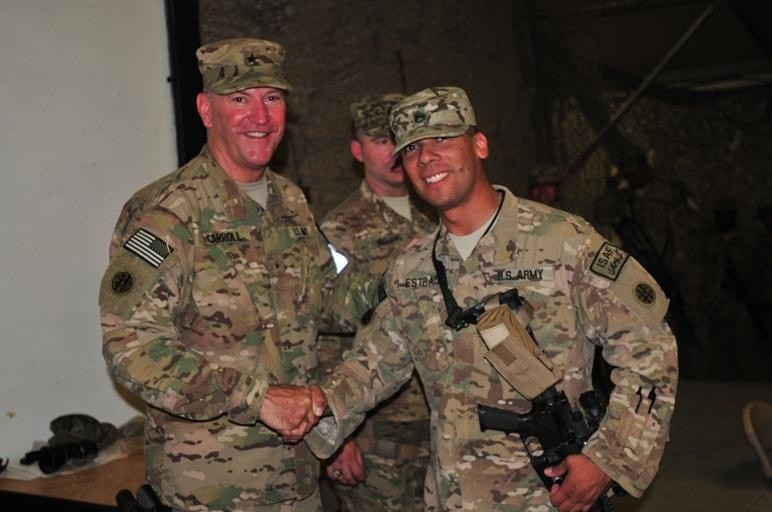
[528,166,559,188]
[390,87,475,159]
[348,93,406,139]
[196,37,292,96]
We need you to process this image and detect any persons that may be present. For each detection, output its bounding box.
[273,85,679,512]
[98,36,390,512]
[522,163,560,206]
[306,93,434,512]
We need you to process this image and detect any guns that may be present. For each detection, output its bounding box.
[478,386,625,510]
[594,216,696,361]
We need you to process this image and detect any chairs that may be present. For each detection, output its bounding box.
[742,400,771,480]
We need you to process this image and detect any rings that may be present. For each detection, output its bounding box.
[331,470,343,478]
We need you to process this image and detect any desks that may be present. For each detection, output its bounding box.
[0,432,144,511]
